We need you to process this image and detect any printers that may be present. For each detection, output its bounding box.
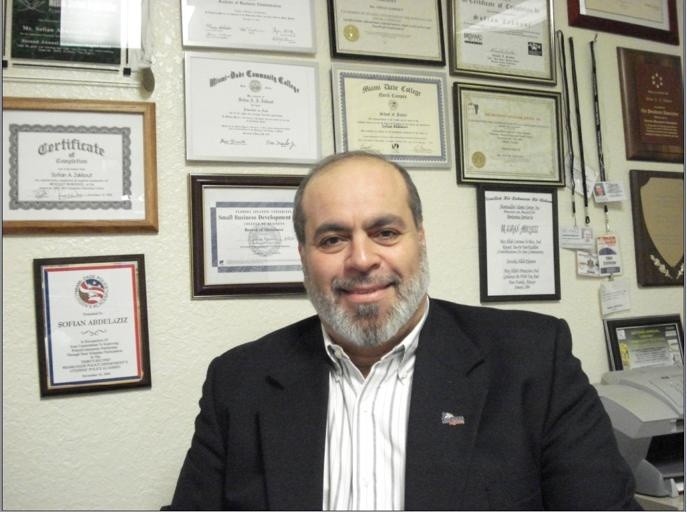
[593,364,684,511]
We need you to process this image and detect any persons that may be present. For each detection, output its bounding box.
[159,151,642,511]
